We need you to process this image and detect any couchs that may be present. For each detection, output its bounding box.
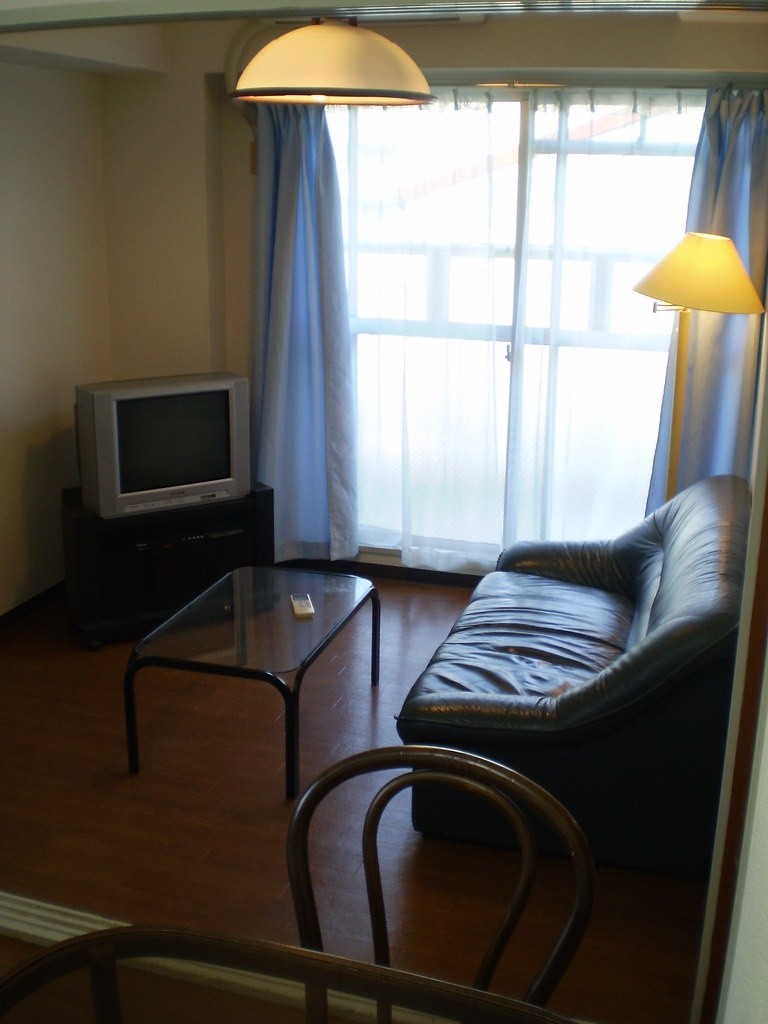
[392,473,757,873]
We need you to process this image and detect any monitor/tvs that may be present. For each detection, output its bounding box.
[73,370,252,518]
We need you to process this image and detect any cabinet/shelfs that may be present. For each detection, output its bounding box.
[59,480,272,650]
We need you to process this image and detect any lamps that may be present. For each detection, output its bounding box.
[628,230,765,503]
[225,11,439,109]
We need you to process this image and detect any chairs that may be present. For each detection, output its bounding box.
[284,739,593,1024]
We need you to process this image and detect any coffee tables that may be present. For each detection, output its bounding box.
[123,564,382,801]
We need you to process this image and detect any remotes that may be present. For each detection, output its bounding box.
[291,591,316,618]
